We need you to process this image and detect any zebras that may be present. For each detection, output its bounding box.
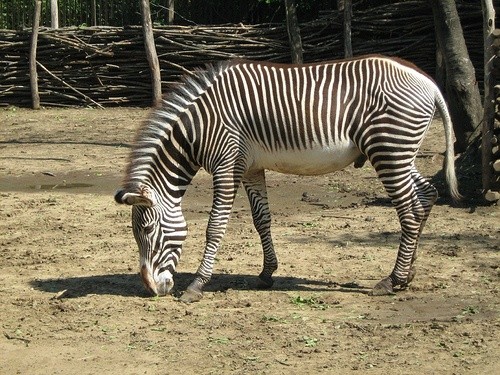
[114,54,463,303]
